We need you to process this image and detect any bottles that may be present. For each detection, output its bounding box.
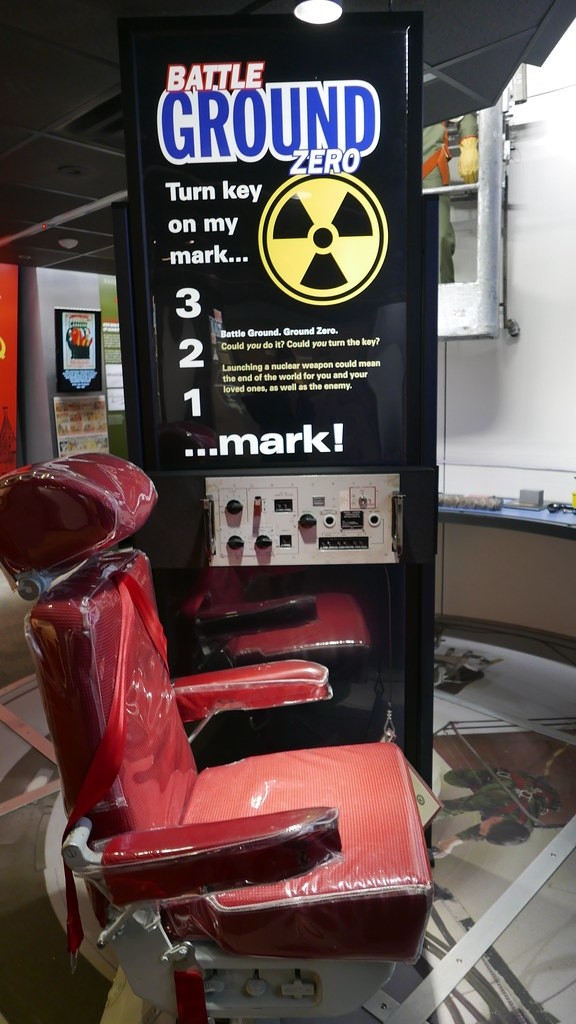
[571,477,576,508]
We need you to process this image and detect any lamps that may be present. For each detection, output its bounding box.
[293,0,344,24]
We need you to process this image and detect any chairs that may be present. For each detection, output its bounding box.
[0,453,434,1024]
[170,527,371,691]
